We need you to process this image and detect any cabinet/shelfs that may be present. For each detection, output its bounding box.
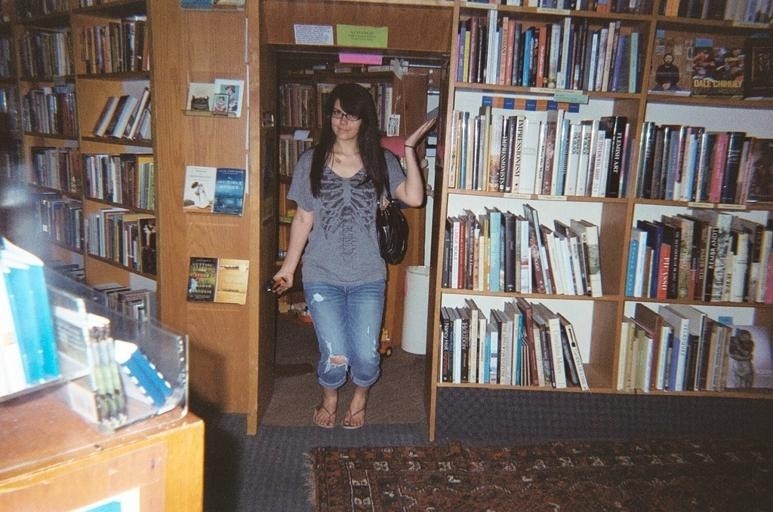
[431,1,772,446]
[277,74,396,351]
[181,0,248,313]
[0,0,163,343]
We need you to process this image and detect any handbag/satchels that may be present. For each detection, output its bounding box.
[376,192,409,265]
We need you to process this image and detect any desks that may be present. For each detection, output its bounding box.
[0,383,208,512]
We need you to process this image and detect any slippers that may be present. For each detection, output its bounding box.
[313,404,337,429]
[340,405,365,430]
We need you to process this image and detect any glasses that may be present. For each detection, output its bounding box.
[332,111,360,121]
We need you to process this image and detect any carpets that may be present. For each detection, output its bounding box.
[307,444,768,511]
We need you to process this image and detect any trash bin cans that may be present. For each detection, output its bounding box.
[401,265,430,355]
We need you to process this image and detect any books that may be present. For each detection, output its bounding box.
[449,92,772,209]
[439,202,602,390]
[276,56,443,310]
[456,1,773,95]
[617,210,773,390]
[1,1,249,430]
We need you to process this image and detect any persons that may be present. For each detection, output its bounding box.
[268,82,438,428]
